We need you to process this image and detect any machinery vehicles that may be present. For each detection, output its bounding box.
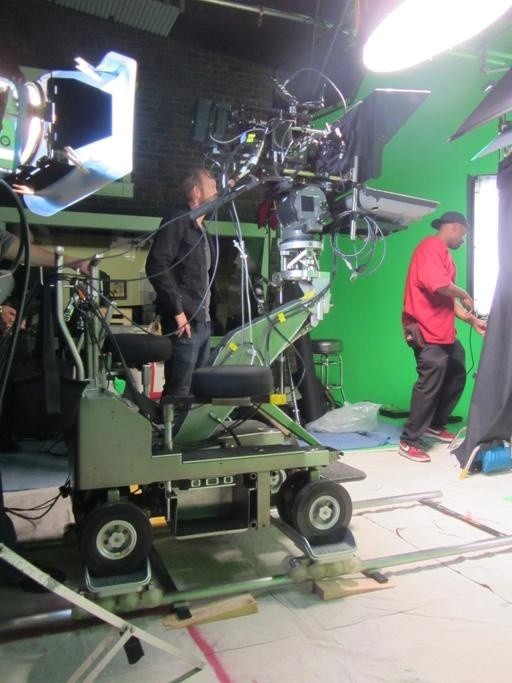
[53,246,366,572]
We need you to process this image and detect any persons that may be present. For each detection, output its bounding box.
[145,165,219,411]
[0,231,92,337]
[398,211,489,461]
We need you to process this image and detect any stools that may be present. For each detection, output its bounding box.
[312,338,344,402]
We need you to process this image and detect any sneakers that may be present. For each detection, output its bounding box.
[423,426,454,442]
[399,439,430,461]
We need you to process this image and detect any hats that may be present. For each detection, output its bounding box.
[432,211,466,228]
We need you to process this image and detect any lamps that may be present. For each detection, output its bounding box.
[354,1,512,78]
